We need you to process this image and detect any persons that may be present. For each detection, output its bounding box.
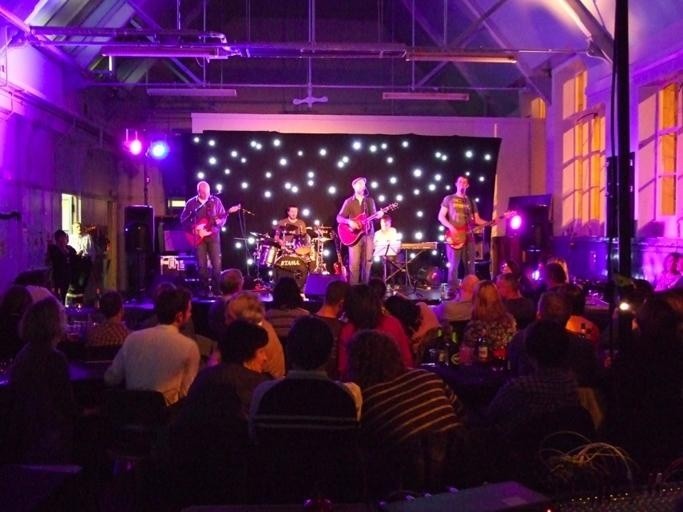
[438,175,496,300]
[1,229,683,511]
[337,177,384,286]
[373,215,400,264]
[181,181,229,300]
[273,206,312,258]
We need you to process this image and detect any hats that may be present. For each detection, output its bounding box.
[351,177,367,186]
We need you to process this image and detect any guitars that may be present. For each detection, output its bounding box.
[338,202,400,246]
[185,205,242,246]
[444,209,518,250]
[332,232,348,283]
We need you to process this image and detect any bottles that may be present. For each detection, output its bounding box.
[92,288,102,308]
[434,324,514,367]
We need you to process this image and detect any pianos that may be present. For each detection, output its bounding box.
[398,242,440,250]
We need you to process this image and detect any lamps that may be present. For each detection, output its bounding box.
[147,132,171,162]
[123,128,143,156]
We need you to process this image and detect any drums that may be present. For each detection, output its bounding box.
[273,255,308,291]
[255,245,282,270]
[292,233,312,254]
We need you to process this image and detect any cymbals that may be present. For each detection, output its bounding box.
[318,226,332,230]
[272,224,298,231]
[312,237,330,241]
[250,232,277,241]
